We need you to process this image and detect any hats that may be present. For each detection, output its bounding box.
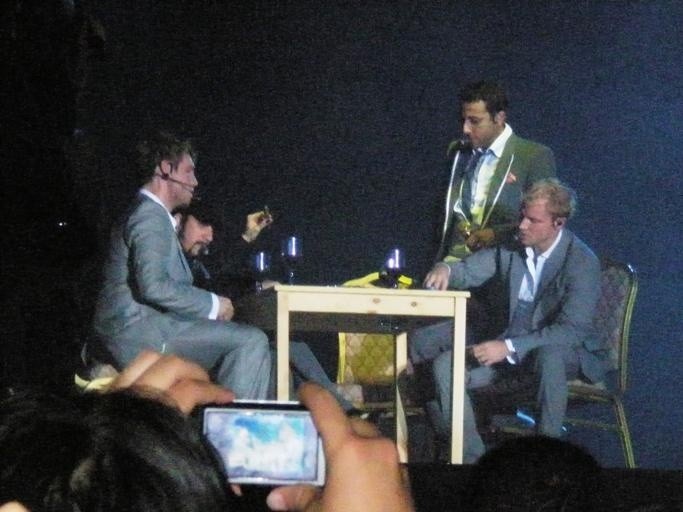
[175,194,223,230]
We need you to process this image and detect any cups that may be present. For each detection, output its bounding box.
[249,250,273,278]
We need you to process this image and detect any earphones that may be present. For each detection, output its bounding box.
[557,220,563,225]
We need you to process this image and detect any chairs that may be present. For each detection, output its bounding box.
[338,261,436,467]
[467,261,639,471]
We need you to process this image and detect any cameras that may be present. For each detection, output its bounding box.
[189,398,327,490]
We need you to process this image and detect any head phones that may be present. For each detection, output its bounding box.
[172,211,185,236]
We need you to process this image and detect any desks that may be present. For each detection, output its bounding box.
[232,285,470,469]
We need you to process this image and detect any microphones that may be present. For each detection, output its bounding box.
[203,246,209,256]
[162,172,202,202]
[458,139,465,147]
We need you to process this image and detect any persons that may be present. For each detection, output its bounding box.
[420,175,621,465]
[455,430,607,512]
[82,127,278,409]
[165,186,390,424]
[425,76,559,272]
[1,339,426,510]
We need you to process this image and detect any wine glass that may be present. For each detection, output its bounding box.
[377,242,412,290]
[279,234,307,285]
